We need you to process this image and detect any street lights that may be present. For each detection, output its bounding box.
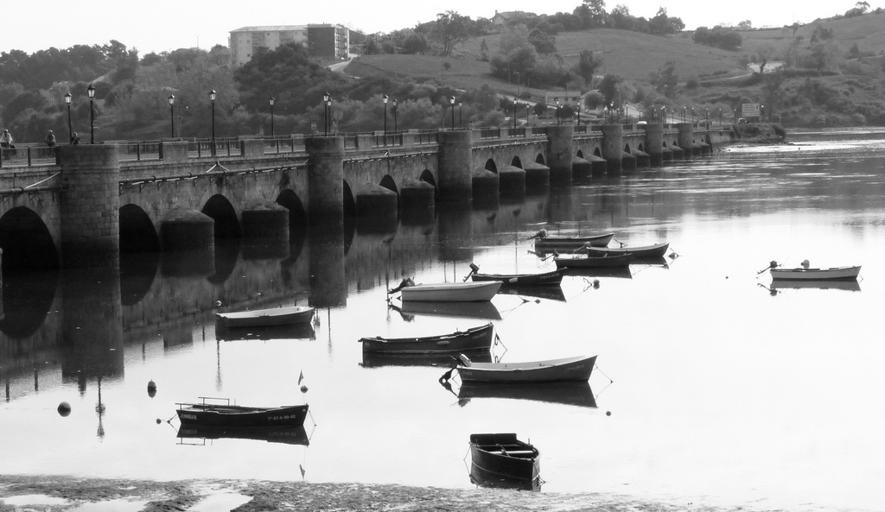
[63,89,73,144]
[87,80,97,142]
[269,95,276,146]
[625,102,629,123]
[207,88,217,155]
[576,100,582,126]
[603,100,615,124]
[449,96,463,132]
[169,93,175,137]
[322,92,333,137]
[381,94,398,148]
[650,103,738,127]
[512,97,530,138]
[556,100,564,127]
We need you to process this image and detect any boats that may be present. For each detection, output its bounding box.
[463,262,568,286]
[213,323,318,346]
[756,256,862,280]
[361,318,495,353]
[757,277,864,295]
[359,348,492,369]
[438,352,600,383]
[177,394,310,427]
[529,229,670,269]
[385,298,503,322]
[496,283,568,303]
[559,264,633,279]
[438,380,598,409]
[215,302,317,327]
[389,277,505,303]
[469,462,544,495]
[174,423,309,448]
[466,431,541,482]
